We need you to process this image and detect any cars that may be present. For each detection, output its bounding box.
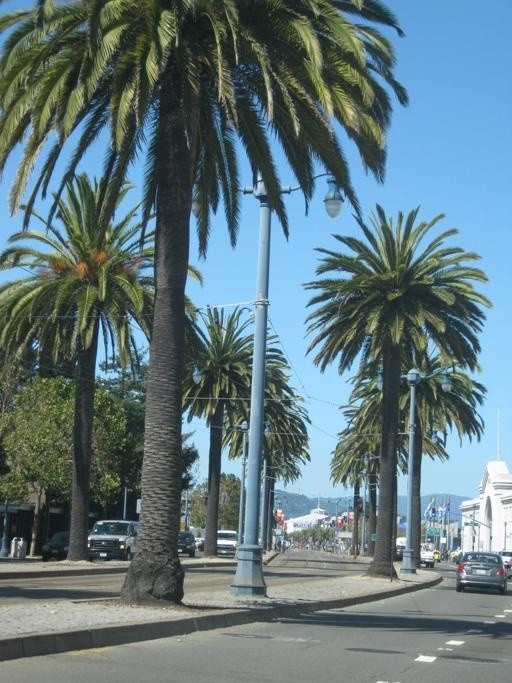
[42,530,73,564]
[457,552,507,594]
[194,537,204,552]
[434,551,440,563]
[498,549,511,578]
[394,545,405,560]
[178,531,195,558]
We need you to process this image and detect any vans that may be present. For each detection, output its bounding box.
[87,519,139,560]
[325,543,333,551]
[217,529,239,557]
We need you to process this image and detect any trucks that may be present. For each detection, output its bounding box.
[419,543,434,569]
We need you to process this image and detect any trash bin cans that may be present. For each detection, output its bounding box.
[11,538,28,560]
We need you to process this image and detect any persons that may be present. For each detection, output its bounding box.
[283,541,286,553]
[445,551,448,562]
[504,561,508,572]
[278,541,281,552]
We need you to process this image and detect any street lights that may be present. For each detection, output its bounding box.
[119,359,140,519]
[184,484,194,530]
[233,419,291,563]
[227,163,347,600]
[397,363,457,575]
[183,352,203,385]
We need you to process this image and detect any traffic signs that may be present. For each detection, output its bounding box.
[427,527,442,538]
[465,522,479,526]
[372,534,376,540]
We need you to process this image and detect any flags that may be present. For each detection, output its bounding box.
[424,496,450,517]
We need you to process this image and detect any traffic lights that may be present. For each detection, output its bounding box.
[432,508,436,516]
